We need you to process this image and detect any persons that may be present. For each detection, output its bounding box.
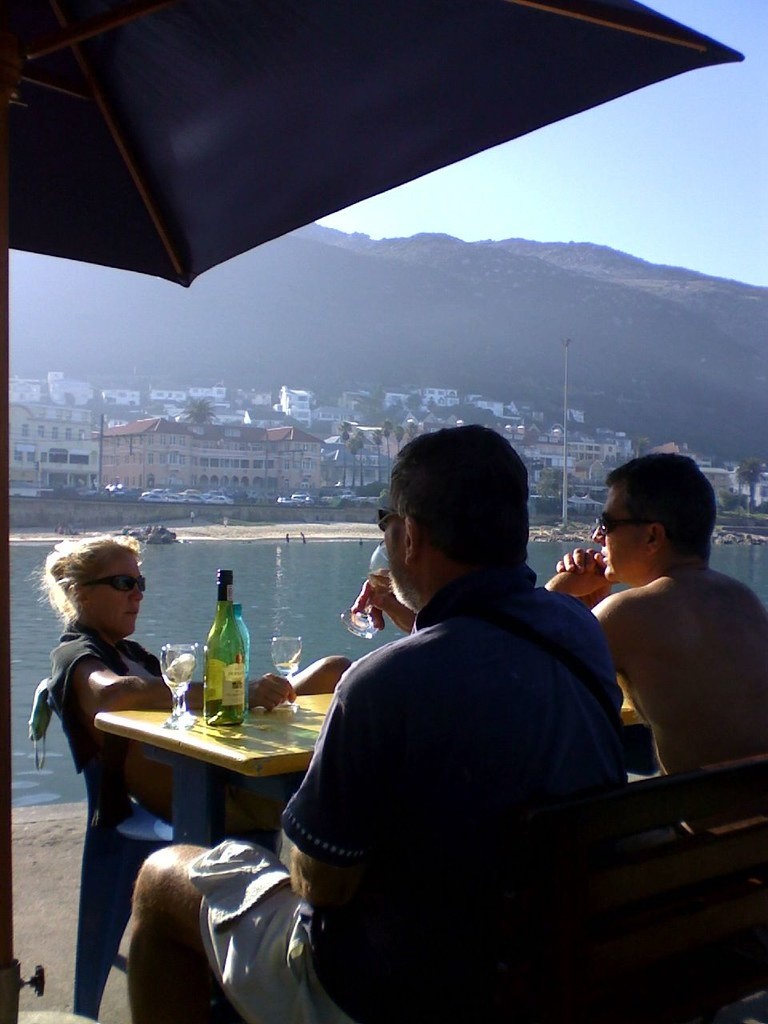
[544,453,767,833]
[127,425,624,1024]
[44,536,353,832]
[141,511,364,547]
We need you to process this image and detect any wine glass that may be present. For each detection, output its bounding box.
[166,644,198,725]
[270,635,303,708]
[159,645,191,730]
[339,540,394,638]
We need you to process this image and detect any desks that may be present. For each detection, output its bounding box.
[94,694,650,847]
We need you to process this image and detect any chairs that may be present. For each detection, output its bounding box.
[32,676,274,1022]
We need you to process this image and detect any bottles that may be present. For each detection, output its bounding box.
[230,603,250,719]
[204,569,247,725]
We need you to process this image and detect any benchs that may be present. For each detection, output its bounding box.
[526,749,768,1023]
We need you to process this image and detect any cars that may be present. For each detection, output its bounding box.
[138,488,234,505]
[277,492,314,507]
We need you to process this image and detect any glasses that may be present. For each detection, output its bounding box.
[600,513,656,534]
[81,573,146,591]
[377,507,433,531]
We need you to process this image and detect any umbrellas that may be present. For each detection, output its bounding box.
[0,0,748,1024]
